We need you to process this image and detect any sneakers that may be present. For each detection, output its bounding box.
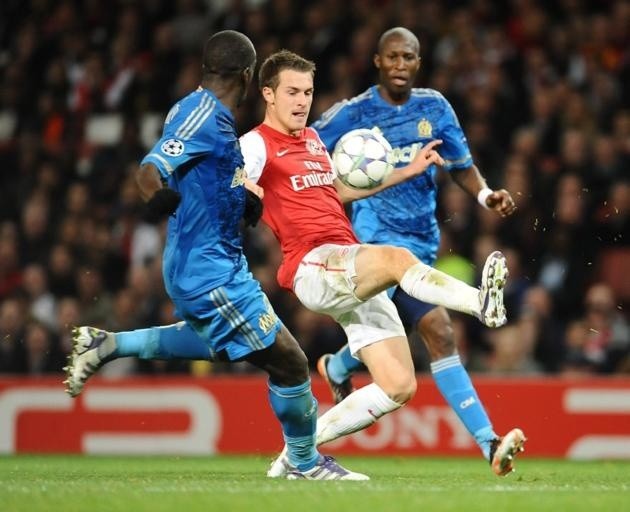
[476,251,510,331]
[266,440,371,482]
[317,350,358,404]
[487,428,528,478]
[62,322,106,398]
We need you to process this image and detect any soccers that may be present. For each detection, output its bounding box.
[331,126,393,191]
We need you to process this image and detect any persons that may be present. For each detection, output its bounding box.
[309,27,526,475]
[64,29,371,481]
[239,51,509,478]
[0,1,629,375]
